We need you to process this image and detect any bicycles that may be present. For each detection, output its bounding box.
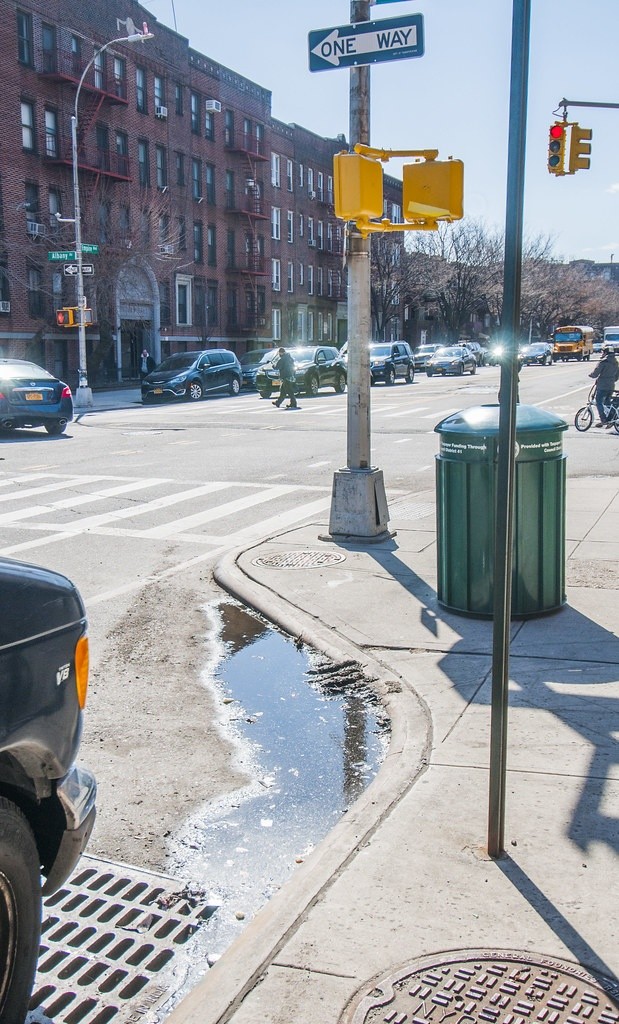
[575,375,619,433]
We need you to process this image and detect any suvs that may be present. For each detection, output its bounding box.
[142,348,244,404]
[370,341,416,385]
[256,346,347,396]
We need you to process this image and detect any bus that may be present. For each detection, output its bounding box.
[550,325,593,362]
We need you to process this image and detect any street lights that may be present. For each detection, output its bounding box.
[71,32,155,405]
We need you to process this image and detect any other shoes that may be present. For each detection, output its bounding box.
[286,404,296,408]
[596,420,608,428]
[272,401,279,407]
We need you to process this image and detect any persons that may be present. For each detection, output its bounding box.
[272,348,297,408]
[138,348,155,383]
[589,346,619,428]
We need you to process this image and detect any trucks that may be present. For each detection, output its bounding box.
[604,326,619,353]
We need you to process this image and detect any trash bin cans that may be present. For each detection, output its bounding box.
[434,404,569,619]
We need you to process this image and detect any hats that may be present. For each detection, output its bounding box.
[601,346,614,354]
[279,348,285,353]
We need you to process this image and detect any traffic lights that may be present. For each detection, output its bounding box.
[569,126,591,171]
[334,151,384,223]
[548,125,566,172]
[57,310,73,325]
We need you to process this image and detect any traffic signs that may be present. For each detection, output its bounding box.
[64,263,94,276]
[307,13,425,73]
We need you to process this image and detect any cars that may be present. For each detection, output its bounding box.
[426,341,501,377]
[0,358,73,436]
[238,348,273,389]
[413,344,444,369]
[522,343,553,365]
[593,343,603,352]
[0,555,97,1024]
